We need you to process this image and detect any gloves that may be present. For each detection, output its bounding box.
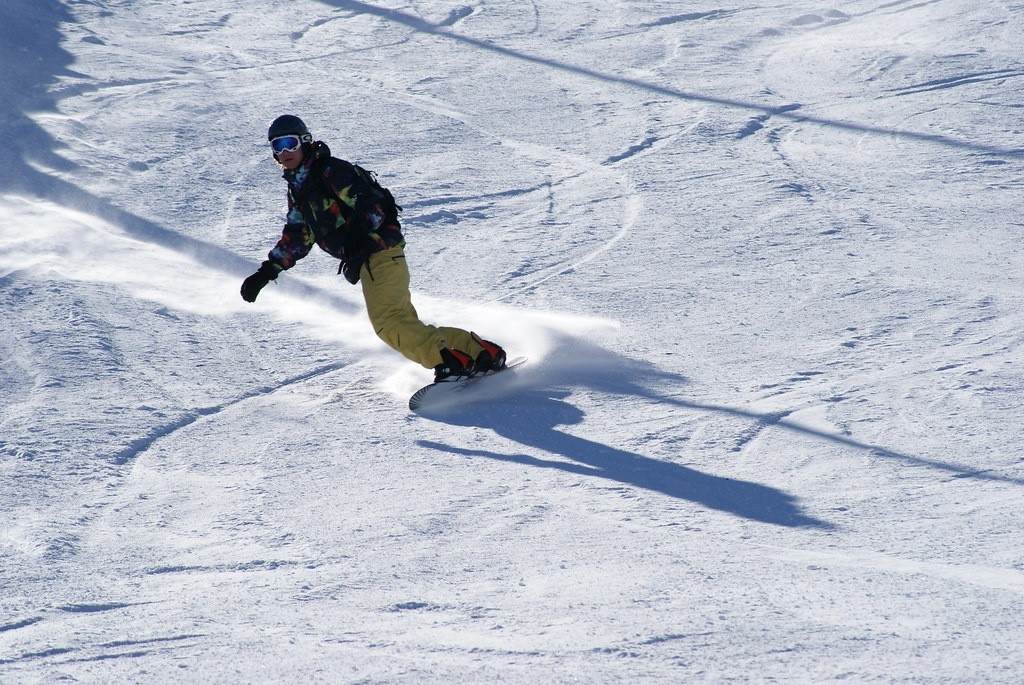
[240,268,269,303]
[344,244,369,284]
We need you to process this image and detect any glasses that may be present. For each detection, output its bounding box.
[270,135,302,155]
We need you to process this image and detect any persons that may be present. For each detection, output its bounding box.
[241,113,507,384]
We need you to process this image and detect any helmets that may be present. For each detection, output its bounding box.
[267,114,308,141]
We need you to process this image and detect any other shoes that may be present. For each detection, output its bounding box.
[434,339,477,383]
[471,331,506,375]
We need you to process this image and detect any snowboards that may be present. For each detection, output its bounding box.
[408,353,528,416]
